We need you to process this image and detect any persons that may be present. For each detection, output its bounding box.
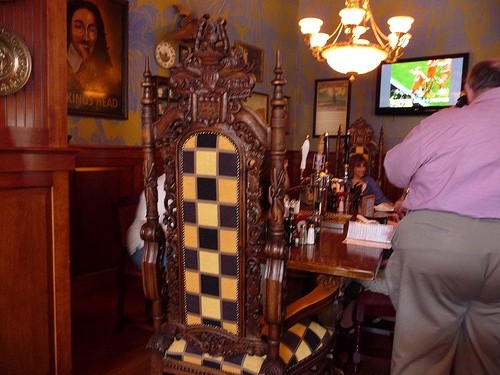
[336,188,409,337]
[348,153,386,202]
[410,58,452,97]
[383,60,500,375]
[127,173,176,271]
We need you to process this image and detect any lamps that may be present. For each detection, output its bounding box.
[298,0,414,82]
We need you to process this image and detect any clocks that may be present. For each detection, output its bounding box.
[154,42,176,68]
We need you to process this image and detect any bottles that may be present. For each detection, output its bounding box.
[284,207,322,247]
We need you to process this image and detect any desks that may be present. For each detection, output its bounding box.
[287,208,384,292]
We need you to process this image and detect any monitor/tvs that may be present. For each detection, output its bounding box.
[375,51,469,114]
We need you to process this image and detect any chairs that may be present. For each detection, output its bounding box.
[351,289,396,375]
[335,117,384,195]
[141,13,346,375]
[112,196,151,335]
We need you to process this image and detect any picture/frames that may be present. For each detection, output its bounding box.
[67,0,130,120]
[233,39,264,83]
[244,91,269,125]
[314,77,351,138]
